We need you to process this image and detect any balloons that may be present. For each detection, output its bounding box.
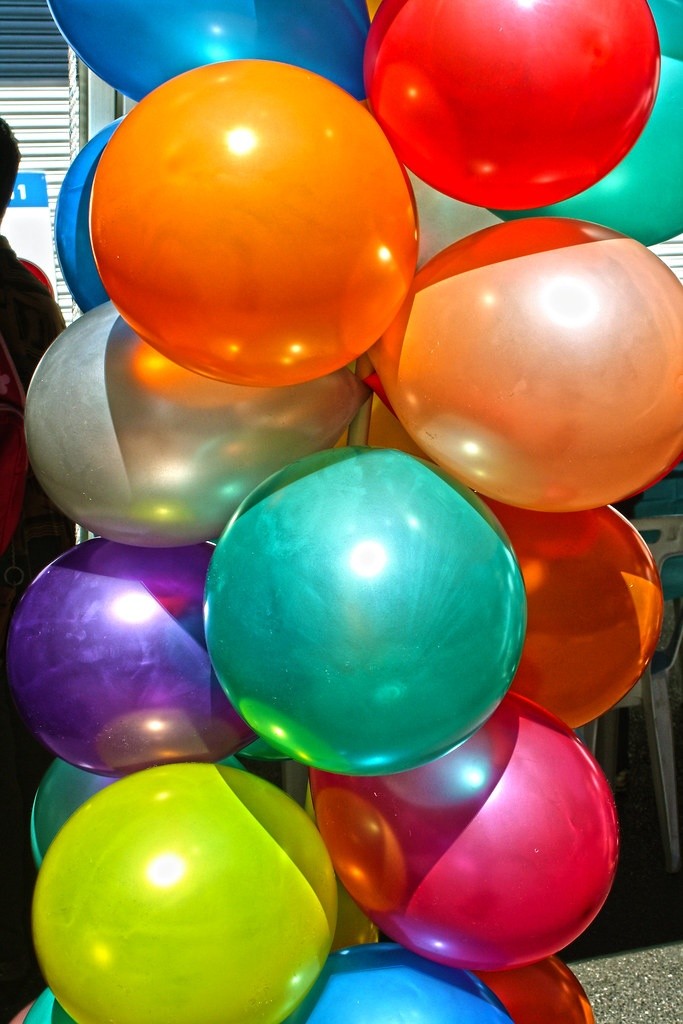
[6,1,683,1023]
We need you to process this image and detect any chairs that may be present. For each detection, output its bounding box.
[584,461,683,873]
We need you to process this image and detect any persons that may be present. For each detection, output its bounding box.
[1,118,78,1024]
